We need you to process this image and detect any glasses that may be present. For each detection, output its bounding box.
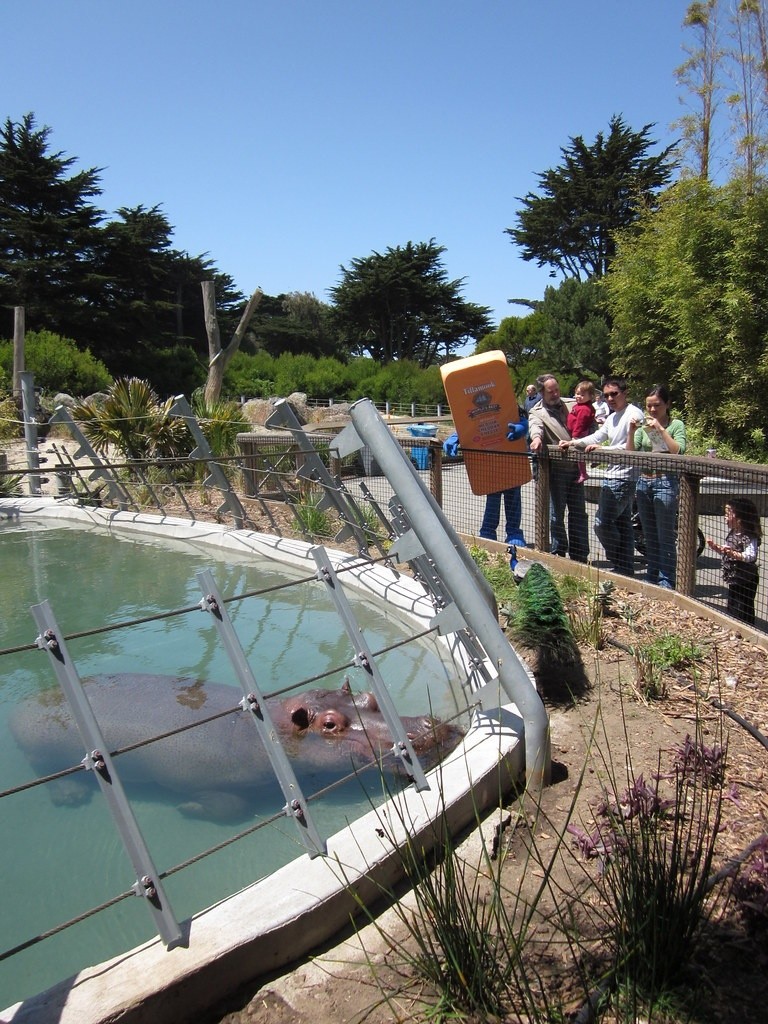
[602,390,624,398]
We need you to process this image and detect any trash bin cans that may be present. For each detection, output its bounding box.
[407,426,439,470]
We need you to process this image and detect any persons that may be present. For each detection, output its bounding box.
[528,374,599,565]
[626,387,686,590]
[707,499,763,625]
[592,394,610,427]
[558,377,647,577]
[525,385,542,414]
[567,381,597,484]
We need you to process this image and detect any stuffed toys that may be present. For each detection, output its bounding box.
[439,350,529,571]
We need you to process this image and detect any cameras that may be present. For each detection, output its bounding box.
[639,418,646,426]
